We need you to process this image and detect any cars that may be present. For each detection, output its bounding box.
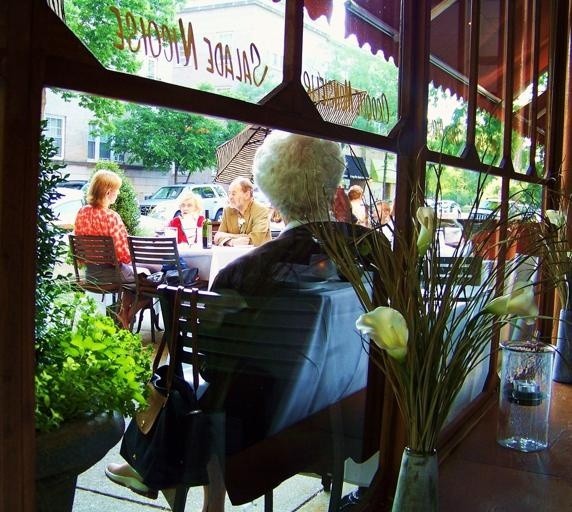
[47,180,141,225]
[388,216,482,287]
[136,182,233,228]
[471,197,533,224]
[423,194,460,217]
[249,185,278,215]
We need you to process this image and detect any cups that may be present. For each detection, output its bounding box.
[165,226,178,246]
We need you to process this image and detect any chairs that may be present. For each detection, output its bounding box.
[64,234,162,336]
[127,234,199,333]
[156,284,373,511]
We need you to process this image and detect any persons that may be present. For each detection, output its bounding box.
[215,177,274,250]
[163,192,208,247]
[374,201,390,228]
[103,125,392,501]
[73,168,149,329]
[342,185,367,227]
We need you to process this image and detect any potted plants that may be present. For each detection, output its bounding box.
[32,118,157,512]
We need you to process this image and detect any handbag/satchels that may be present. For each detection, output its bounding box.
[120,366,209,491]
[148,255,200,286]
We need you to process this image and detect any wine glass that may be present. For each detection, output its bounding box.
[154,221,165,238]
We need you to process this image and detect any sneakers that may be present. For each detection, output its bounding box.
[106,462,158,500]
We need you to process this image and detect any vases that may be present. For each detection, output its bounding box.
[554,308,572,385]
[393,450,443,512]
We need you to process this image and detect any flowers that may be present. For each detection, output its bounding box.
[284,148,559,454]
[527,198,570,314]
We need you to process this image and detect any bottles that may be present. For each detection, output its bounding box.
[495,339,558,453]
[200,209,213,249]
